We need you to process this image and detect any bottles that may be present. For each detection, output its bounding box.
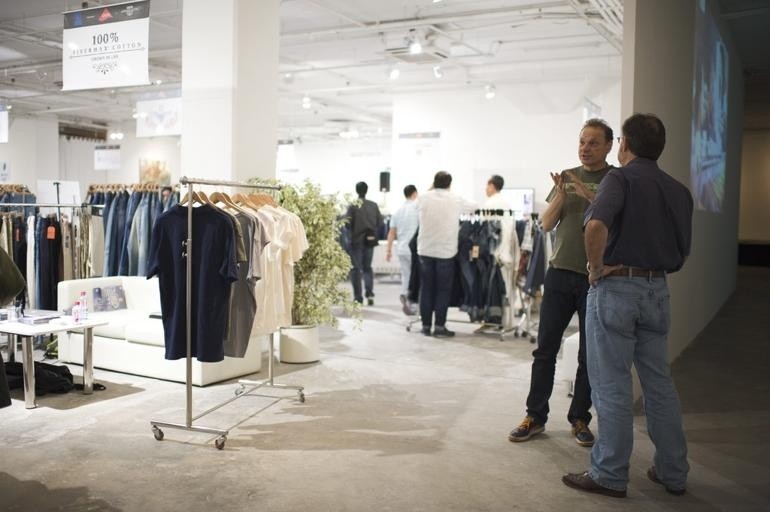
[79,291,88,320]
[72,301,83,325]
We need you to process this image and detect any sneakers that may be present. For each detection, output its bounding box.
[400,293,417,315]
[434,327,455,338]
[572,419,595,446]
[508,415,545,442]
[420,327,431,336]
[367,296,374,306]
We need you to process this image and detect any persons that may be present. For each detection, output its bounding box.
[344,182,386,307]
[415,166,482,340]
[506,117,614,447]
[385,184,420,316]
[560,110,695,500]
[481,173,512,211]
[160,187,172,201]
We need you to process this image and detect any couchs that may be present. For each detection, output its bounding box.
[52,268,271,388]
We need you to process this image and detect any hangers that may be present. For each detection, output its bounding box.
[1,204,92,219]
[171,179,282,213]
[459,208,518,226]
[0,182,34,196]
[86,182,169,194]
[520,211,545,231]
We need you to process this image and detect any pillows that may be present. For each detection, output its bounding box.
[90,284,128,313]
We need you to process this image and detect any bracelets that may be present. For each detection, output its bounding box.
[588,262,602,275]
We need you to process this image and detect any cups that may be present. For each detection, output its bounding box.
[7,306,22,321]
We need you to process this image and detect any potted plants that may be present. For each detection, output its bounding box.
[250,176,377,366]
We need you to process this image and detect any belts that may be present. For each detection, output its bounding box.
[607,267,664,277]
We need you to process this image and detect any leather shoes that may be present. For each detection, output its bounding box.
[561,471,626,498]
[646,466,685,495]
